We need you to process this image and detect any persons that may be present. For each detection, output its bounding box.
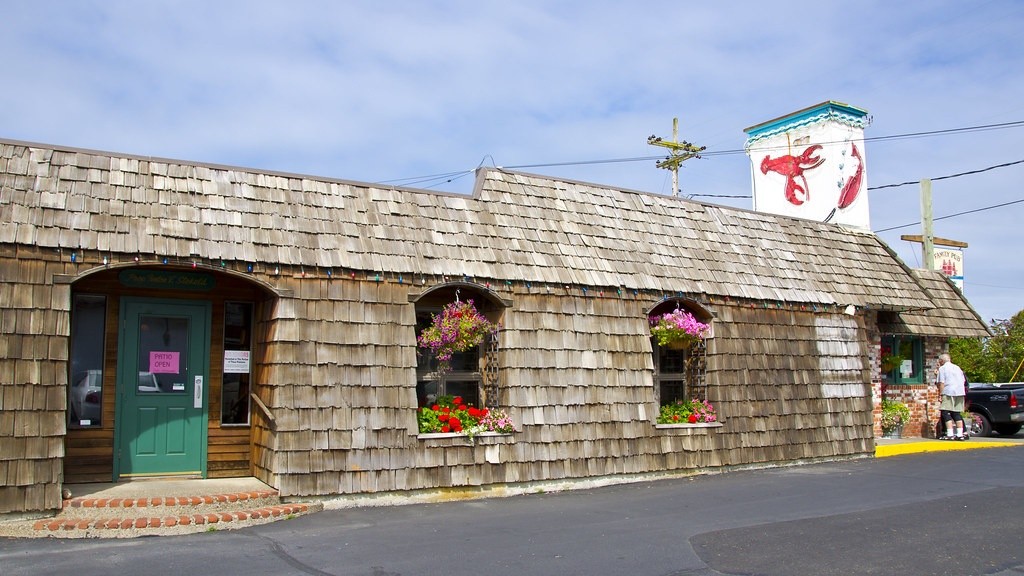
[935,354,969,440]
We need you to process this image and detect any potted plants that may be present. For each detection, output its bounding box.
[881,399,911,439]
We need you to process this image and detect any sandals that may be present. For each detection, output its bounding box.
[954,436,965,440]
[963,433,969,440]
[939,435,955,440]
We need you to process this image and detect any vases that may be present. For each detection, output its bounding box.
[883,423,902,439]
[962,418,973,437]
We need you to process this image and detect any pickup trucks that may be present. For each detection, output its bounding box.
[964,382,1024,437]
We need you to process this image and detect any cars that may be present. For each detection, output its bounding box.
[70,368,103,426]
[138,368,164,393]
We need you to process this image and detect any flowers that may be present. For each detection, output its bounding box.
[960,410,982,426]
[880,346,906,370]
[881,379,890,396]
[648,308,711,346]
[417,298,503,376]
[416,394,515,445]
[656,397,719,424]
